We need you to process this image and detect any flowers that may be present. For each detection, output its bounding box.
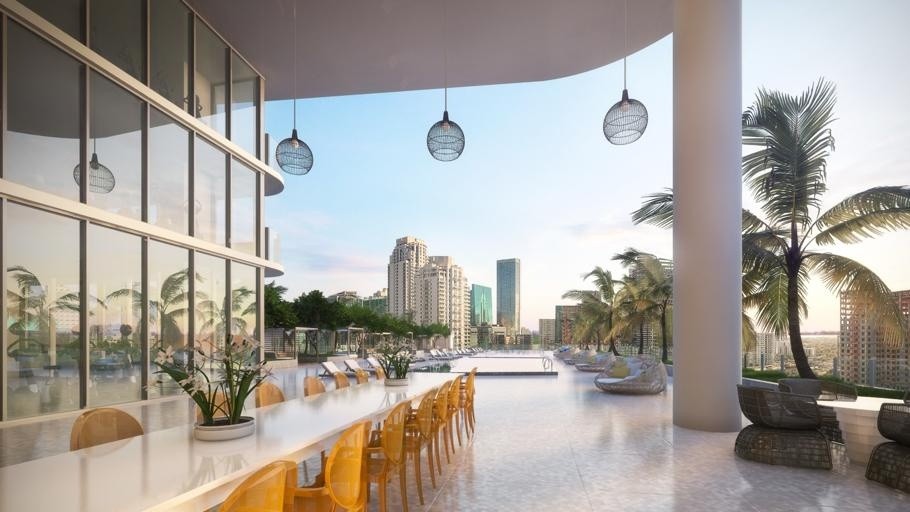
[148,340,274,421]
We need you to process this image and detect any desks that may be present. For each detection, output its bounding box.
[23,369,468,512]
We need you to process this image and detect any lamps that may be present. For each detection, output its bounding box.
[427,0,471,162]
[603,1,649,149]
[75,105,116,193]
[275,0,313,176]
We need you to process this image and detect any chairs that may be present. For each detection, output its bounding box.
[318,345,489,365]
